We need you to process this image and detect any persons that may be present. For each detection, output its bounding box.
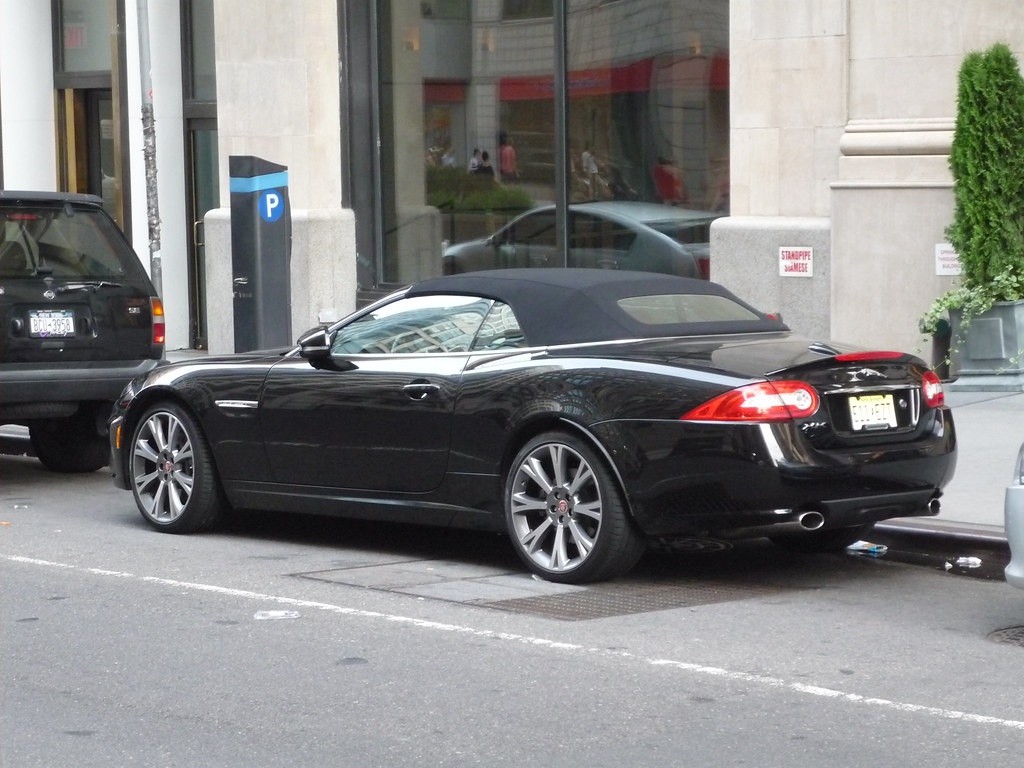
[477,151,494,173]
[501,138,520,181]
[468,148,482,171]
[655,154,687,205]
[571,143,614,200]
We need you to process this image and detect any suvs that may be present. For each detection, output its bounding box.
[0,190,166,474]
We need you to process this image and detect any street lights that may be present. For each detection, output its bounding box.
[588,108,598,154]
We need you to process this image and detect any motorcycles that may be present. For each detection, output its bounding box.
[575,174,640,203]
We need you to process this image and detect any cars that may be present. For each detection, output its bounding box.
[440,201,731,281]
[107,268,956,585]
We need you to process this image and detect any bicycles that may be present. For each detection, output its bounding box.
[599,153,641,188]
